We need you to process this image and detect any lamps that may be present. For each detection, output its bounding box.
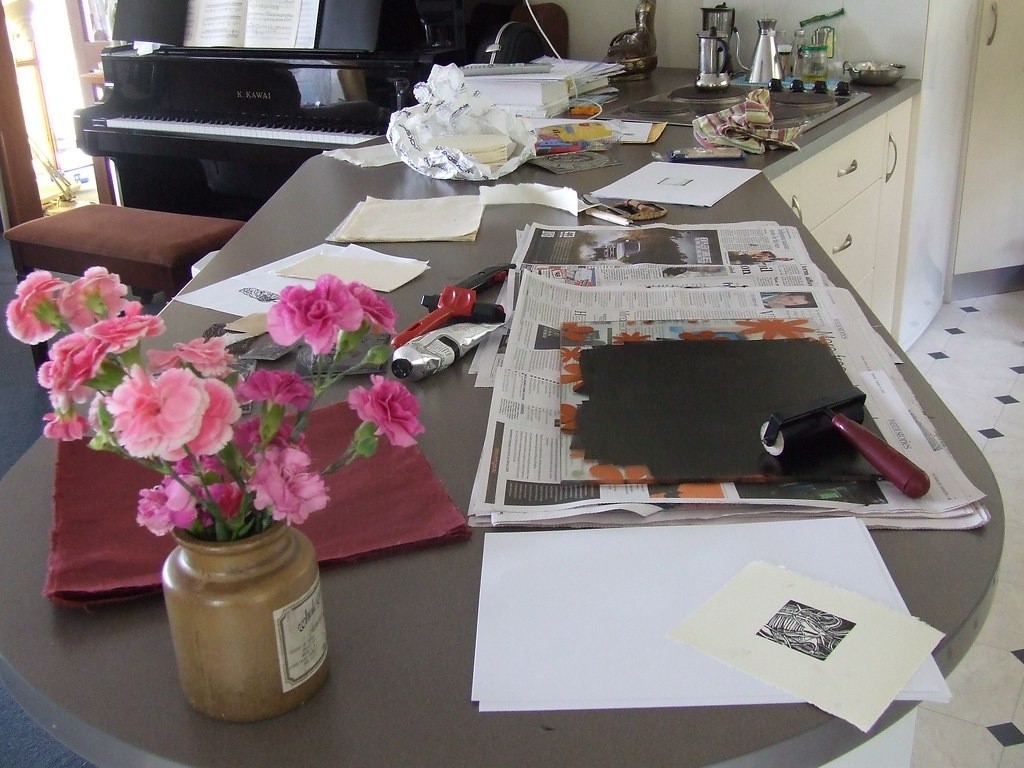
[4,0,81,216]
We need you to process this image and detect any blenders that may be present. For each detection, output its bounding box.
[700,2,736,78]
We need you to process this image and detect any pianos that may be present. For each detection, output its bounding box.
[71,1,515,222]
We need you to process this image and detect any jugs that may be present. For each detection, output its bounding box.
[695,28,730,90]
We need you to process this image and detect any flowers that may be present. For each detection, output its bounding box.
[4,266,425,544]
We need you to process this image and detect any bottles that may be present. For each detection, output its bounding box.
[776,27,834,81]
[749,20,782,90]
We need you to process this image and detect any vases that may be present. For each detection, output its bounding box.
[161,521,331,724]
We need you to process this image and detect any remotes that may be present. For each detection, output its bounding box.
[462,63,551,75]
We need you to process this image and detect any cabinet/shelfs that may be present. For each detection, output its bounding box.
[770,97,912,335]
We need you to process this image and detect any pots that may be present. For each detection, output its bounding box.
[842,59,905,87]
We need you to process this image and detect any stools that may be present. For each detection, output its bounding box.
[3,201,248,390]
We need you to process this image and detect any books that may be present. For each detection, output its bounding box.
[571,76,609,97]
[183,0,320,48]
[461,63,569,117]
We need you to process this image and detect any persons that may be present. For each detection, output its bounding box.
[729,251,775,265]
[761,292,812,308]
